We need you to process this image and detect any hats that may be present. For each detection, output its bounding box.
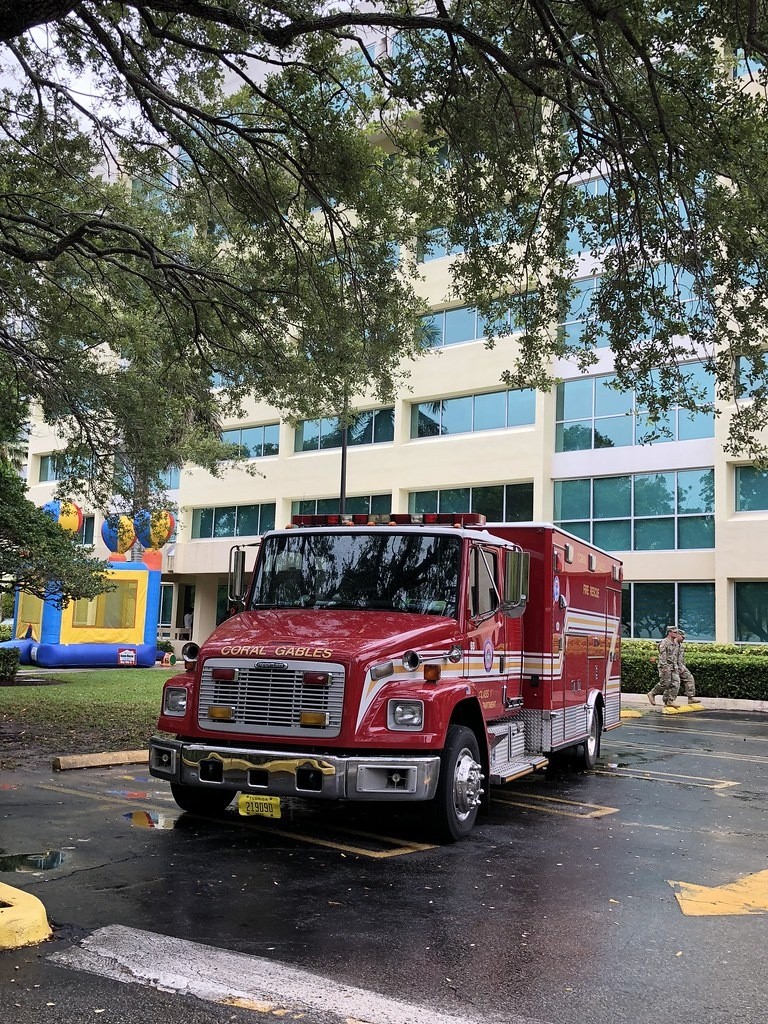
[677,629,686,637]
[666,626,678,632]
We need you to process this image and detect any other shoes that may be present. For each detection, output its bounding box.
[647,692,656,705]
[688,697,701,704]
[666,700,681,708]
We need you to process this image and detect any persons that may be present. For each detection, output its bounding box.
[647,626,701,708]
[216,610,231,628]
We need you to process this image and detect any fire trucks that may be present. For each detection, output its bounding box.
[147,510,627,845]
